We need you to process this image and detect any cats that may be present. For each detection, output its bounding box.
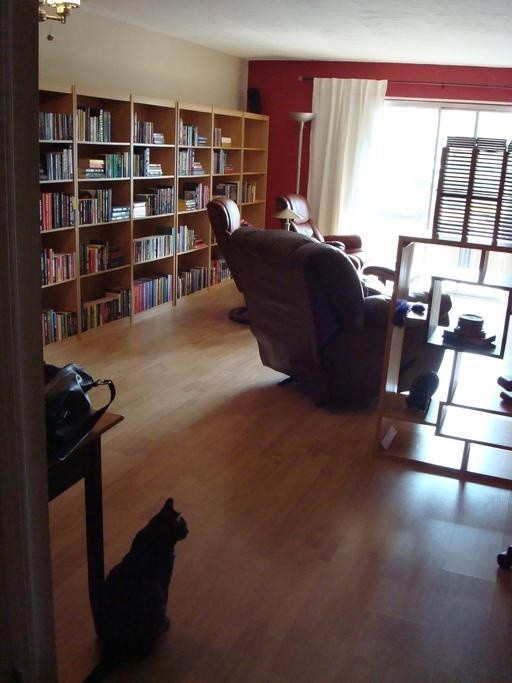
[83,498,189,683]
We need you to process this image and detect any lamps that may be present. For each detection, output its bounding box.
[37,1,83,42]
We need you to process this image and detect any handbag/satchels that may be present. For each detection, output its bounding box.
[44,360,115,462]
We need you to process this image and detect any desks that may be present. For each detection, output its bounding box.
[47,411,126,641]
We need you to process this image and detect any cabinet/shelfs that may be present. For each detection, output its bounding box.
[371,138,511,491]
[39,78,270,353]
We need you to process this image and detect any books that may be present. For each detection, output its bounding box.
[131,224,173,314]
[40,107,132,232]
[442,334,492,348]
[178,116,256,212]
[133,111,175,217]
[41,237,132,344]
[443,328,497,346]
[444,337,496,350]
[175,225,233,299]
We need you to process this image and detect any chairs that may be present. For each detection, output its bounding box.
[276,194,363,272]
[208,196,353,322]
[227,227,400,403]
